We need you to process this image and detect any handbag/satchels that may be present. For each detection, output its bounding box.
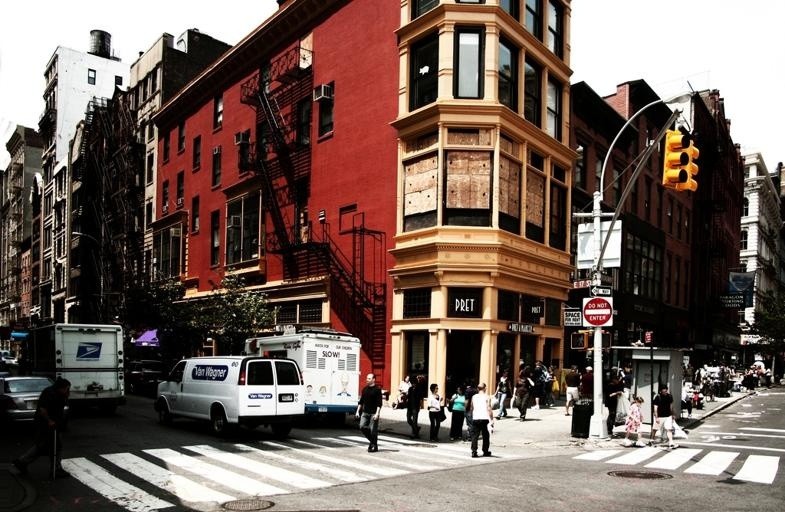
[440,408,446,423]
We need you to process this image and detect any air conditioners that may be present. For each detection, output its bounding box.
[213,147,220,154]
[170,228,181,237]
[311,83,331,101]
[234,130,249,145]
[228,215,242,227]
[177,198,183,204]
[163,205,168,211]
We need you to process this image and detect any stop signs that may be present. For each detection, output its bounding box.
[583,296,615,326]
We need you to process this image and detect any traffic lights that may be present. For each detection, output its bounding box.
[663,130,691,190]
[686,138,699,192]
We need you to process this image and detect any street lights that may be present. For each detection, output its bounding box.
[69,231,104,321]
[593,87,692,438]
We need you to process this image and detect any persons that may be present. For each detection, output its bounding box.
[336,374,351,397]
[649,385,680,449]
[396,361,646,458]
[354,373,382,453]
[681,364,776,418]
[10,375,71,483]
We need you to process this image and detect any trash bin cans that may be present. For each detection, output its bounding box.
[571,399,594,439]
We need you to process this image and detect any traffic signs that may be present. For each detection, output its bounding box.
[590,286,612,296]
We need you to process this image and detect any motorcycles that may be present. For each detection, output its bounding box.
[683,362,716,411]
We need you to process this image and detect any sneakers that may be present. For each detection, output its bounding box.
[368,446,378,452]
[471,451,491,457]
[621,437,679,450]
